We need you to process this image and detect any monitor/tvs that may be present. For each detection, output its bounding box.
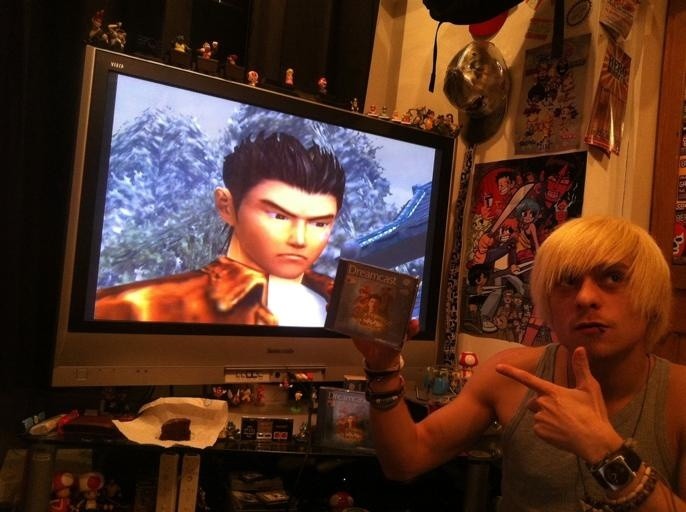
[51,45,459,387]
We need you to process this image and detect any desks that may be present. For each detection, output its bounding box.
[63,417,497,512]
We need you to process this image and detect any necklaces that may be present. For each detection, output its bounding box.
[566,351,651,511]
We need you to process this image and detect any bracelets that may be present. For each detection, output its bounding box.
[608,466,658,511]
[365,376,406,409]
[362,353,405,380]
[604,466,651,505]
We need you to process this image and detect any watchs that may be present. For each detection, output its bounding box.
[588,441,642,491]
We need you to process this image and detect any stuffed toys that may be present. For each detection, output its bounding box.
[47,471,75,511]
[73,471,105,512]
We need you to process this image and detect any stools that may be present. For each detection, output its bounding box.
[19,430,105,512]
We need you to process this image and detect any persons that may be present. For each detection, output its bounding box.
[351,98,461,139]
[168,34,258,87]
[286,68,326,96]
[91,124,347,333]
[84,8,461,141]
[464,153,584,349]
[343,412,359,433]
[86,9,127,51]
[354,286,368,313]
[324,214,685,512]
[359,294,382,328]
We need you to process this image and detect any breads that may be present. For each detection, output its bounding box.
[160,418,191,441]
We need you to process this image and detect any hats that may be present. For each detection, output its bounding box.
[469,11,507,39]
[444,41,510,145]
